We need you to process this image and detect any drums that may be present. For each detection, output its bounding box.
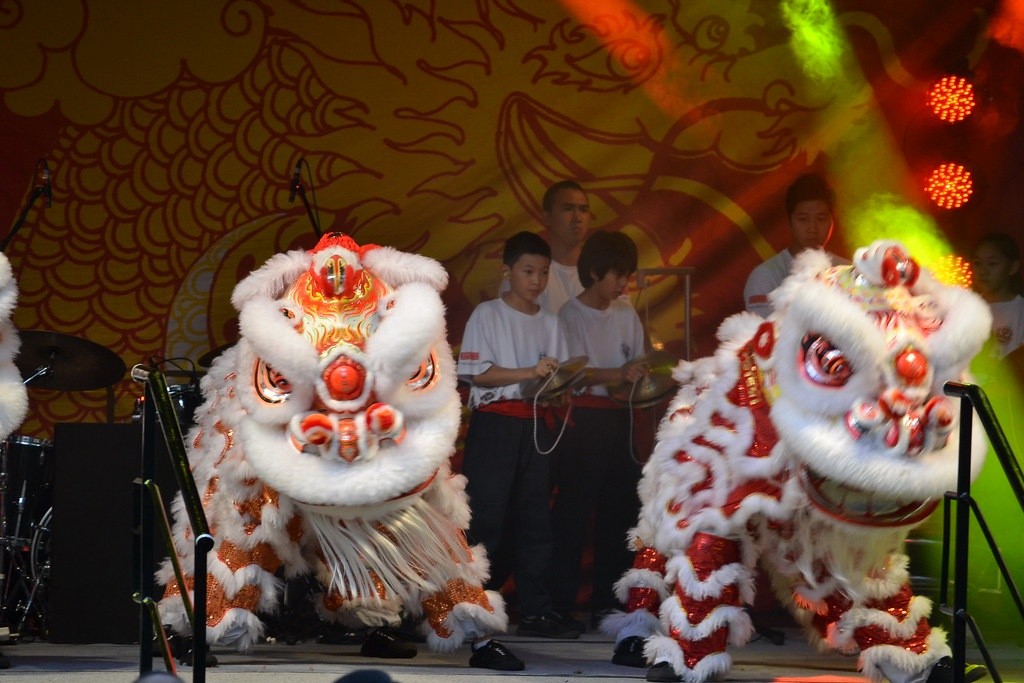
[1,432,55,545]
[27,503,56,594]
[130,383,201,481]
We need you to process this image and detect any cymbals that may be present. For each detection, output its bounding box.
[11,327,126,392]
[196,339,237,369]
[520,355,591,402]
[606,349,679,409]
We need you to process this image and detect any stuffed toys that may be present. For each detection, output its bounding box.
[152,232,524,671]
[0,254,28,439]
[602,242,993,683]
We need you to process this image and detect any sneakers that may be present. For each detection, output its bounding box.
[612,636,646,667]
[645,662,681,682]
[517,613,579,639]
[927,656,987,683]
[468,640,524,671]
[360,627,417,658]
[552,614,586,634]
[180,643,217,667]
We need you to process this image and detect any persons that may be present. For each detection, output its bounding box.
[972,234,1024,393]
[457,180,645,639]
[744,173,852,319]
